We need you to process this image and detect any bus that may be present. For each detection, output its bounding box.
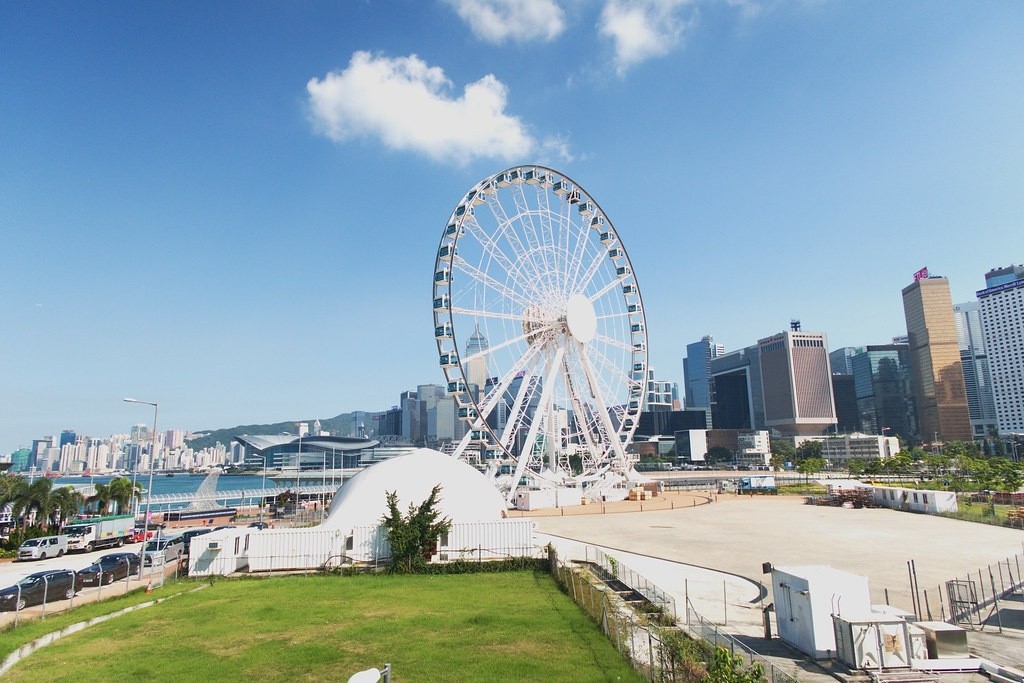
[80,515,101,520]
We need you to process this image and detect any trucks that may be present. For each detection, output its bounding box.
[64,515,137,553]
[738,477,776,494]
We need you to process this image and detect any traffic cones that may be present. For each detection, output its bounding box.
[145,580,153,594]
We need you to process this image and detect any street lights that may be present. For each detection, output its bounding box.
[252,453,267,521]
[123,395,159,531]
[935,431,939,454]
[1015,444,1022,462]
[283,432,303,517]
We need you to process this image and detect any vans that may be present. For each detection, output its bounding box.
[138,535,184,565]
[18,535,66,561]
[183,529,211,554]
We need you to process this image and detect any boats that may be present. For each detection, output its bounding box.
[81,474,91,478]
[166,472,175,478]
[121,472,131,476]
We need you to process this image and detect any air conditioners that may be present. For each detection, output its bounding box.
[208,541,223,550]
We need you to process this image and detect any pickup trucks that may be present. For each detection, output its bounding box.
[127,529,153,544]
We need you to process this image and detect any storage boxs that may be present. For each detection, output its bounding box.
[629,484,658,500]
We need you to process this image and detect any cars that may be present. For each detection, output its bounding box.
[247,522,269,530]
[727,460,774,472]
[0,569,84,612]
[214,526,237,533]
[76,552,143,584]
[136,520,167,531]
[668,462,713,471]
